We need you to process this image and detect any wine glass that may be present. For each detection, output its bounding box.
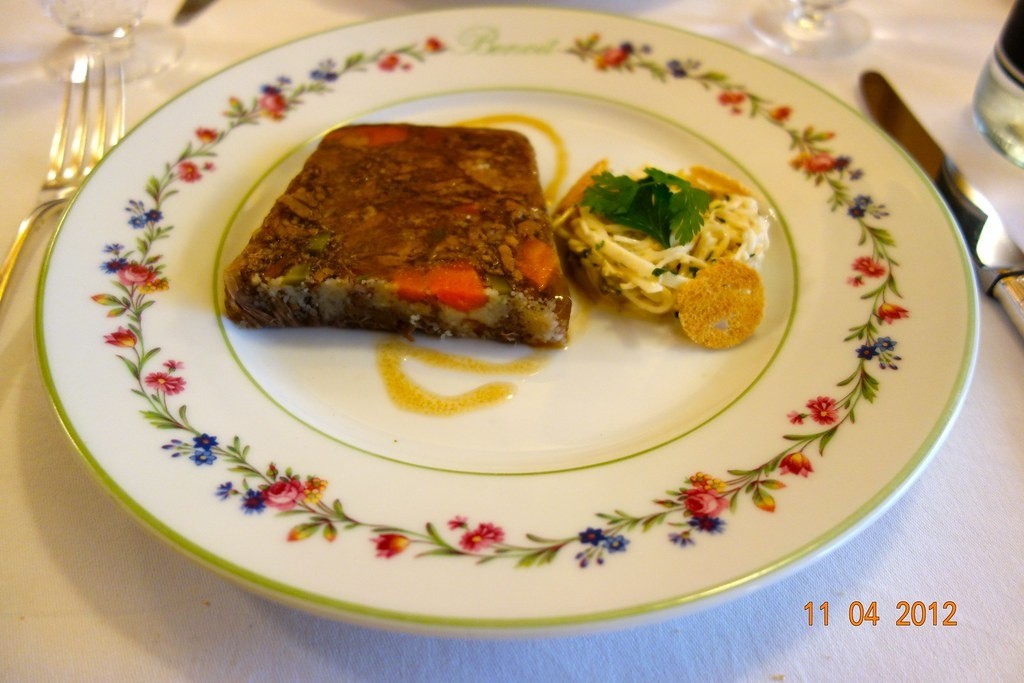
[40,0,183,88]
[744,0,873,60]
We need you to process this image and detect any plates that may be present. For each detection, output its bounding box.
[34,7,981,635]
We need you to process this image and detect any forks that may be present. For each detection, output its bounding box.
[0,55,125,305]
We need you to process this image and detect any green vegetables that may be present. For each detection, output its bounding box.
[573,164,710,249]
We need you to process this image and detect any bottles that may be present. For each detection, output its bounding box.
[971,0,1024,168]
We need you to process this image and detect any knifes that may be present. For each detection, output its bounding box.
[172,0,217,28]
[860,71,1024,348]
